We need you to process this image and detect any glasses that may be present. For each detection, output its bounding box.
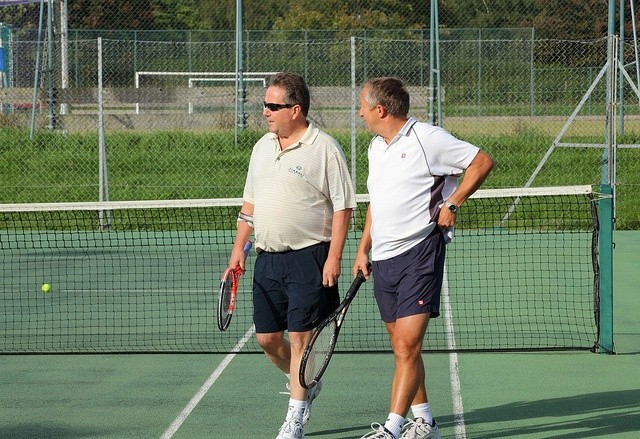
[263,100,295,112]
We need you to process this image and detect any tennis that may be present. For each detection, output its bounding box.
[41,284,51,293]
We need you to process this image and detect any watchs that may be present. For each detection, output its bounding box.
[444,201,458,214]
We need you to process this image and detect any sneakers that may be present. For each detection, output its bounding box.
[358,422,396,439]
[279,377,322,426]
[398,417,441,439]
[275,404,305,439]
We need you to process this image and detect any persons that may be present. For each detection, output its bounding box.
[229,73,357,438]
[353,76,494,438]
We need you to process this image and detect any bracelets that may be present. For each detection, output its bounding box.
[237,212,253,231]
